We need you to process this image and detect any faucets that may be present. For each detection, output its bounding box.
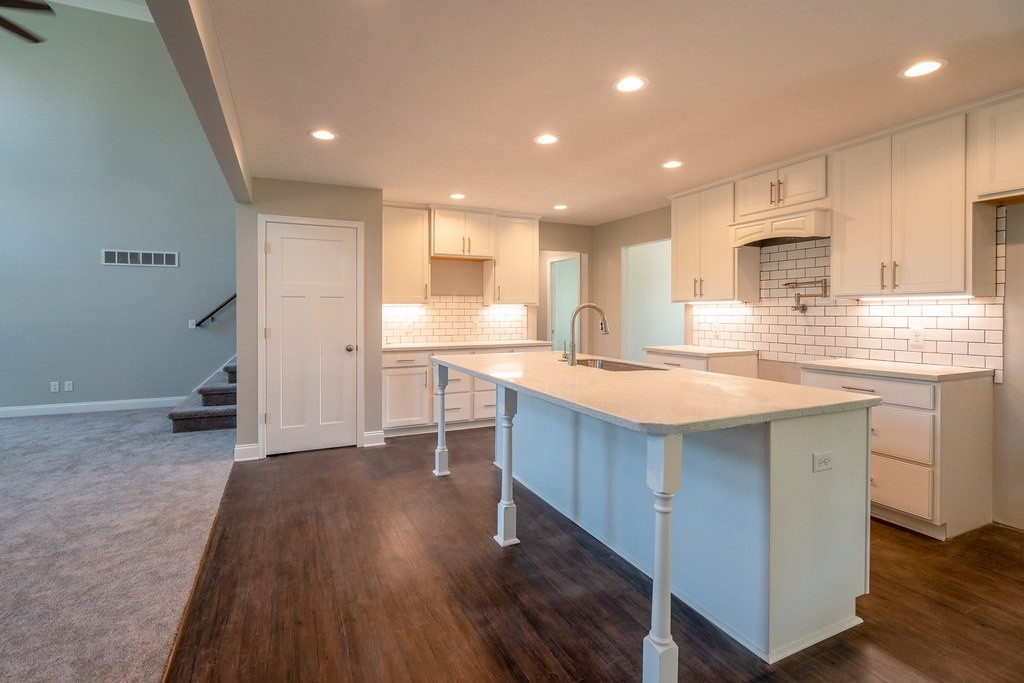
[566,302,610,366]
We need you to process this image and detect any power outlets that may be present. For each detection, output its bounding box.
[64,380,74,392]
[812,450,834,473]
[50,382,60,393]
[188,319,196,329]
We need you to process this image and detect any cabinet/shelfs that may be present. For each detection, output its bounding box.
[641,343,760,377]
[381,346,433,438]
[799,356,996,541]
[667,177,760,304]
[729,146,830,246]
[830,106,997,298]
[381,201,432,303]
[472,341,555,429]
[973,85,1024,206]
[431,346,474,434]
[430,203,495,261]
[482,212,540,306]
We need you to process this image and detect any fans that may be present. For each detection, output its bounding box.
[0,0,52,42]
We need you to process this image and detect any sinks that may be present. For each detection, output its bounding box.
[558,358,670,374]
